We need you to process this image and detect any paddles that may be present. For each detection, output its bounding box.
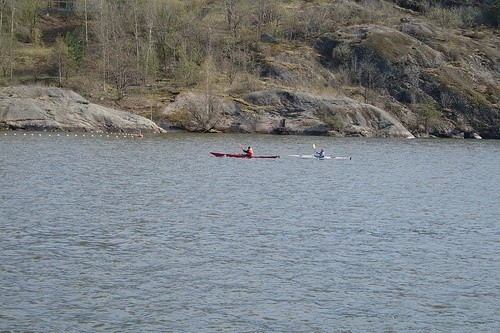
[312,143,319,155]
[238,142,246,156]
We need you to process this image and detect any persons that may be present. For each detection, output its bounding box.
[241,146,253,156]
[314,148,325,158]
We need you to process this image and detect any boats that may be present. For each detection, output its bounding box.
[210,152,280,158]
[289,154,351,159]
